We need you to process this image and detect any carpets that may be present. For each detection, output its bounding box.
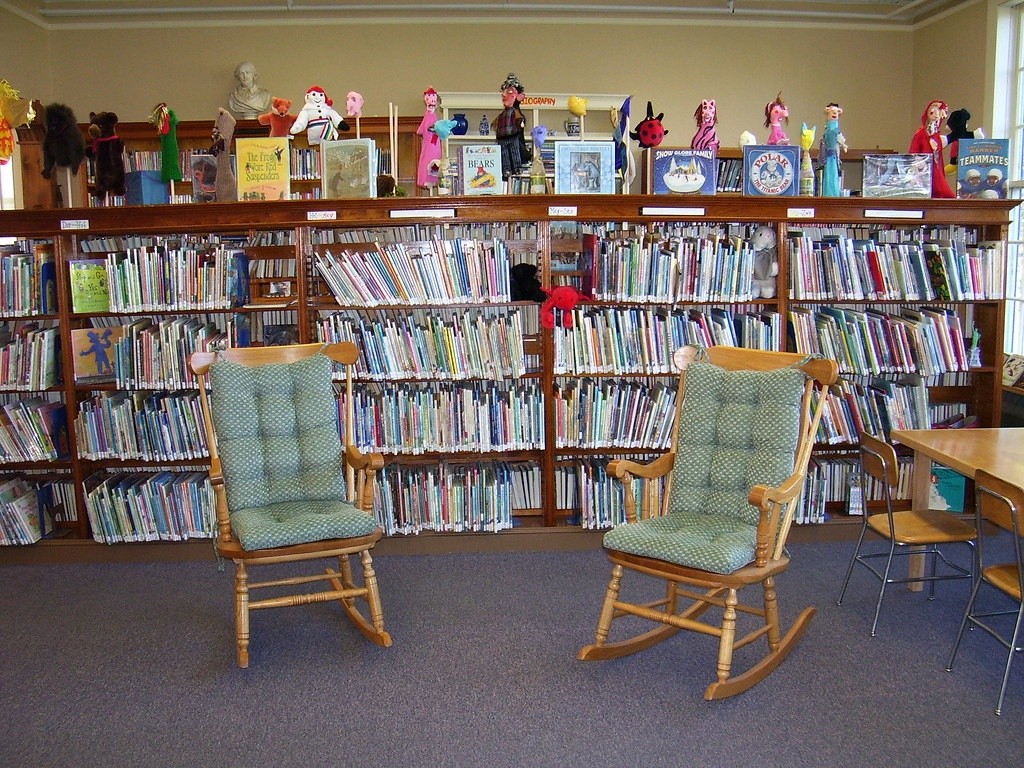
[1,527,1023,768]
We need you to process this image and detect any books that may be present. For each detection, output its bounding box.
[81,465,221,545]
[332,377,547,455]
[716,158,744,192]
[353,459,545,537]
[552,375,680,450]
[87,137,378,207]
[72,388,220,462]
[307,221,542,305]
[309,304,540,382]
[788,222,1006,525]
[62,311,300,391]
[507,141,555,195]
[68,230,301,313]
[550,222,779,305]
[553,305,782,376]
[553,453,673,530]
[0,238,78,546]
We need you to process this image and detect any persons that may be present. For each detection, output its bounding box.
[228,61,270,119]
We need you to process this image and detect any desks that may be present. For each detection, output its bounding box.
[890,428,1024,592]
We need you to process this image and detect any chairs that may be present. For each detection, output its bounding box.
[946,469,1024,718]
[188,341,393,667]
[838,432,980,637]
[575,343,837,700]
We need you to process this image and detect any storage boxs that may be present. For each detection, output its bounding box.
[957,139,1010,199]
[860,150,933,198]
[123,169,168,205]
[743,146,800,198]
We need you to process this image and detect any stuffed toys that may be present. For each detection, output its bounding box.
[259,86,351,146]
[540,285,594,329]
[629,100,669,149]
[751,226,779,299]
[931,107,984,176]
[88,110,127,199]
[0,78,84,180]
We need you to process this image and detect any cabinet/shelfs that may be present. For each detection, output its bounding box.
[1,90,1024,545]
